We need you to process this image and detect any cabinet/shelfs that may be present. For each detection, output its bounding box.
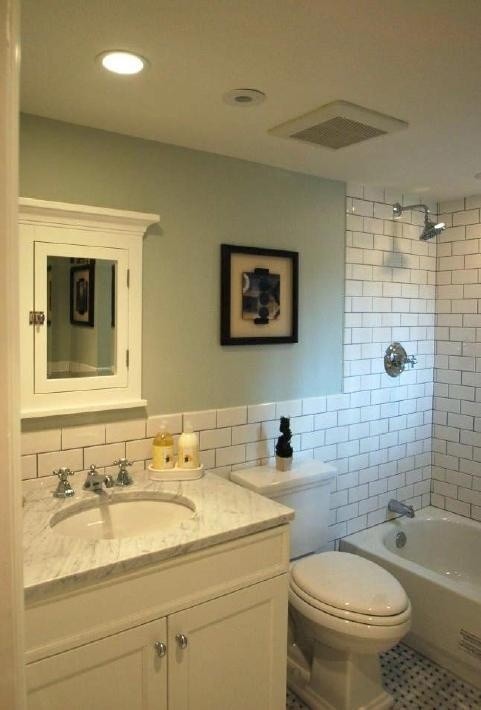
[24,570,292,710]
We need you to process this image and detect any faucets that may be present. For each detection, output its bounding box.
[385,497,419,518]
[82,464,115,492]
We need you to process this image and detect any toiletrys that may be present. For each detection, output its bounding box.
[152,417,176,470]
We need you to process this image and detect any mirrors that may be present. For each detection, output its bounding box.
[33,240,131,396]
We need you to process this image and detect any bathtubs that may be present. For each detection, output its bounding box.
[339,503,481,689]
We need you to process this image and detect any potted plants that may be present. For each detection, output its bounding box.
[275,415,295,473]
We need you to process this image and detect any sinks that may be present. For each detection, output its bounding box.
[44,487,201,546]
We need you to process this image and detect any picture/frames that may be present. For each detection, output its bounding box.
[219,241,301,348]
[69,262,96,328]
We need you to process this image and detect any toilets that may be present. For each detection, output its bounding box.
[228,458,414,710]
